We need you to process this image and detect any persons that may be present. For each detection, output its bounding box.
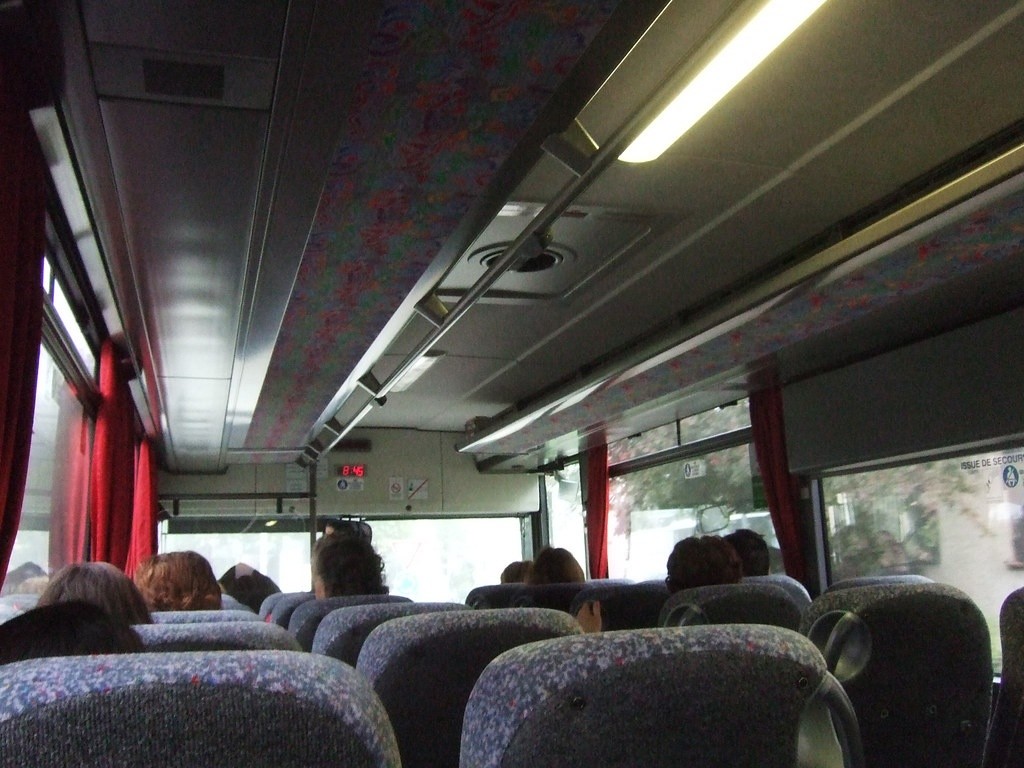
[309,527,390,601]
[136,551,224,612]
[501,529,771,634]
[0,559,154,666]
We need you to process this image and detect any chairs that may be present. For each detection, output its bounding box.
[2,574,1024,768]
